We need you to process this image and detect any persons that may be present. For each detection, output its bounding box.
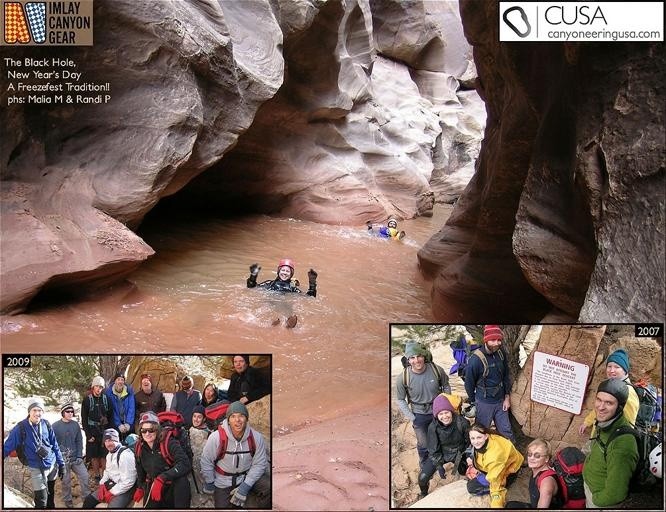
[247,259,317,330]
[366,219,405,242]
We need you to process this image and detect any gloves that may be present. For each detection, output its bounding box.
[365,219,373,226]
[58,465,67,481]
[134,488,145,503]
[150,476,164,500]
[399,230,405,240]
[104,490,113,503]
[307,268,317,284]
[249,261,260,276]
[229,481,252,506]
[199,484,215,505]
[98,484,106,500]
[437,464,446,480]
[119,423,130,433]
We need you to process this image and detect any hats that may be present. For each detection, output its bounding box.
[91,374,153,388]
[432,396,454,417]
[102,428,119,442]
[405,339,425,359]
[192,406,206,417]
[61,401,74,412]
[182,375,194,390]
[139,411,160,425]
[226,402,249,422]
[232,354,250,366]
[28,397,45,413]
[596,378,629,407]
[606,349,629,373]
[481,325,504,342]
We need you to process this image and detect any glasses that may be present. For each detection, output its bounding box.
[527,452,542,458]
[65,410,73,413]
[141,427,156,433]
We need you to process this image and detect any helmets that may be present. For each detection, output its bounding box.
[388,218,398,229]
[279,259,294,271]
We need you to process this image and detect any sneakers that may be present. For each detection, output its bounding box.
[65,500,73,508]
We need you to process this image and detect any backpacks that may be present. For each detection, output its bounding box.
[401,350,443,404]
[535,447,586,509]
[134,411,194,468]
[16,419,53,465]
[604,424,662,508]
[205,399,256,476]
[452,336,506,385]
[629,377,662,431]
